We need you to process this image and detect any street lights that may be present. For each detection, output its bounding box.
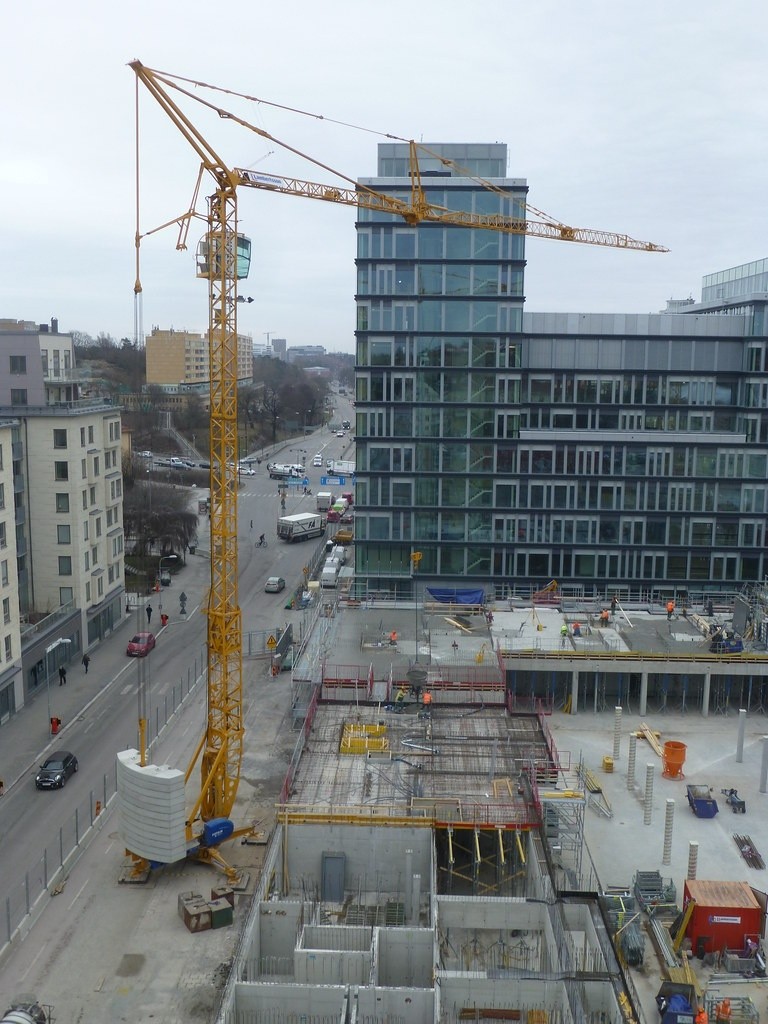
[290,448,304,491]
[146,470,152,511]
[44,638,71,739]
[155,554,177,625]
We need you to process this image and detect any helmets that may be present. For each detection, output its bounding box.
[724,1000,730,1006]
[698,1008,704,1012]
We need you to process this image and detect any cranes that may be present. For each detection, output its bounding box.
[114,58,672,901]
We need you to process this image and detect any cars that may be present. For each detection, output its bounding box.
[160,457,196,472]
[126,632,157,658]
[198,461,217,470]
[225,462,256,476]
[239,457,257,464]
[264,576,286,593]
[35,751,79,791]
[137,451,153,458]
[313,452,324,467]
[336,430,344,437]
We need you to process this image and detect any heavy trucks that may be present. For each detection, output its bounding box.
[276,512,327,543]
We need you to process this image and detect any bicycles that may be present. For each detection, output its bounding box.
[255,539,268,549]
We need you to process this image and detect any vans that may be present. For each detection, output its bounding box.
[320,544,349,591]
[326,492,354,523]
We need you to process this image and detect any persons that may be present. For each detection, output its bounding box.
[601,608,608,627]
[82,654,90,674]
[715,998,731,1024]
[146,604,152,623]
[59,666,66,686]
[259,533,265,545]
[560,624,567,636]
[395,686,408,707]
[573,622,580,636]
[695,1008,709,1024]
[666,600,675,620]
[421,690,432,711]
[389,631,397,645]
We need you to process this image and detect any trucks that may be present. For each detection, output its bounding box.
[266,462,306,480]
[315,492,332,512]
[342,420,350,430]
